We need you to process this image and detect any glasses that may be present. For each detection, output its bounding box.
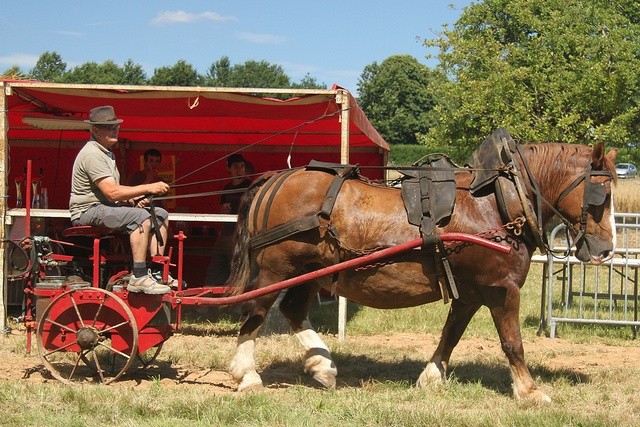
[107,125,120,130]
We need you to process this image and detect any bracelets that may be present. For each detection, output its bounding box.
[127,200,132,207]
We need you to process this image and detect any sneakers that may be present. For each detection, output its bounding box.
[151,269,187,290]
[187,304,207,317]
[126,269,171,294]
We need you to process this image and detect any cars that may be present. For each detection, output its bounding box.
[614,163,638,179]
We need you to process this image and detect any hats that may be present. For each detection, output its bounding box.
[83,106,123,125]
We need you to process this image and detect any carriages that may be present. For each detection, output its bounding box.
[13,128,618,405]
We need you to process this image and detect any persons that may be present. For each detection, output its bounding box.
[68,105,188,296]
[242,161,255,180]
[191,153,260,315]
[125,147,167,207]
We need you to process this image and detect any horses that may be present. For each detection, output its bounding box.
[220,139,619,403]
